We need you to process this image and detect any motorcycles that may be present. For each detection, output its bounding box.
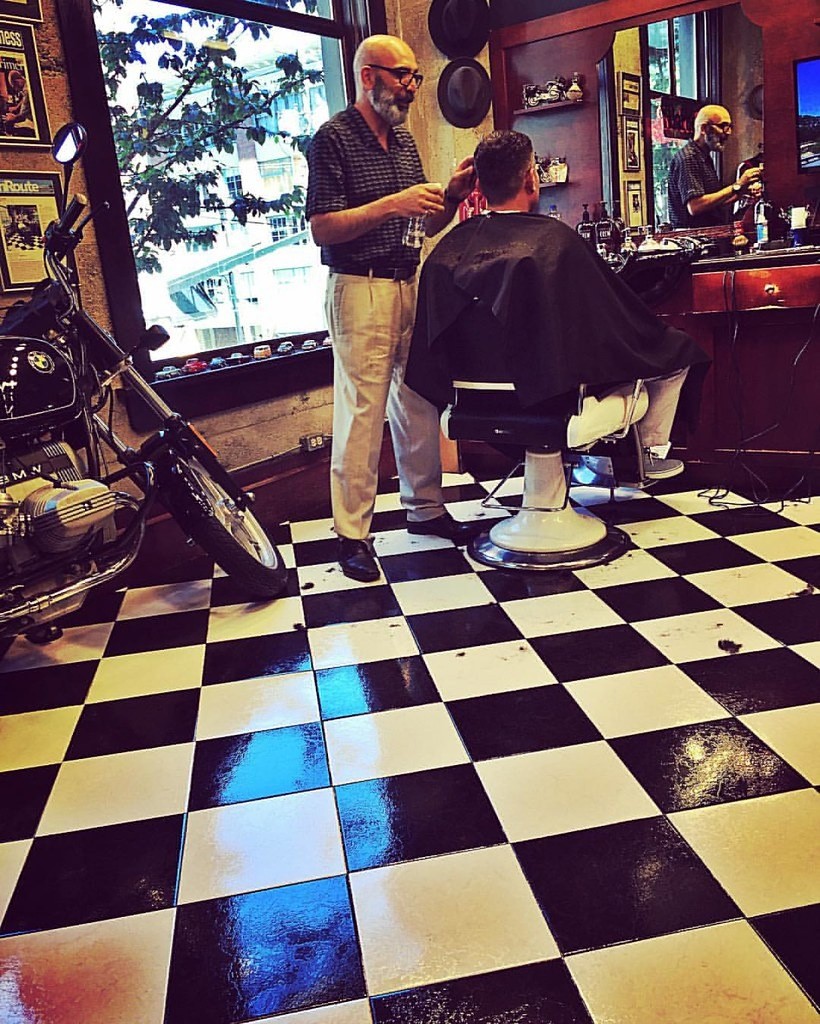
[0,119,290,638]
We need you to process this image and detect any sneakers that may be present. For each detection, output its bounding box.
[643,454,685,480]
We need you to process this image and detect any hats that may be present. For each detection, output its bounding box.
[437,57,490,128]
[427,0,490,61]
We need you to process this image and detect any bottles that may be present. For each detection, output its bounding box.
[547,204,562,222]
[733,232,742,256]
[756,204,770,244]
[567,81,583,101]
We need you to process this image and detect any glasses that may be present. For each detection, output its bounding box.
[710,122,735,133]
[367,64,424,89]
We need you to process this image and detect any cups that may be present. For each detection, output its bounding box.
[791,207,811,246]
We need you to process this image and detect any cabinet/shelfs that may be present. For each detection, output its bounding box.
[640,244,820,485]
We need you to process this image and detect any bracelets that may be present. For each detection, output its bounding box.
[442,189,463,205]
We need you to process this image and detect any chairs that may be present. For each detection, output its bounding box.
[441,371,682,570]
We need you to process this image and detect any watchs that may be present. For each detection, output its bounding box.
[732,182,742,194]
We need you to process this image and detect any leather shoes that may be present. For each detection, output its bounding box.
[407,513,483,541]
[336,533,380,582]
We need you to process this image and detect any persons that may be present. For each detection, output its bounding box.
[306,35,477,581]
[669,106,765,229]
[2,70,36,136]
[419,128,693,478]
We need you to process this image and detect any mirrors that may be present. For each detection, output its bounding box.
[608,3,763,235]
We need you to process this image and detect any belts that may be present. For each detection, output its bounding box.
[329,262,417,282]
